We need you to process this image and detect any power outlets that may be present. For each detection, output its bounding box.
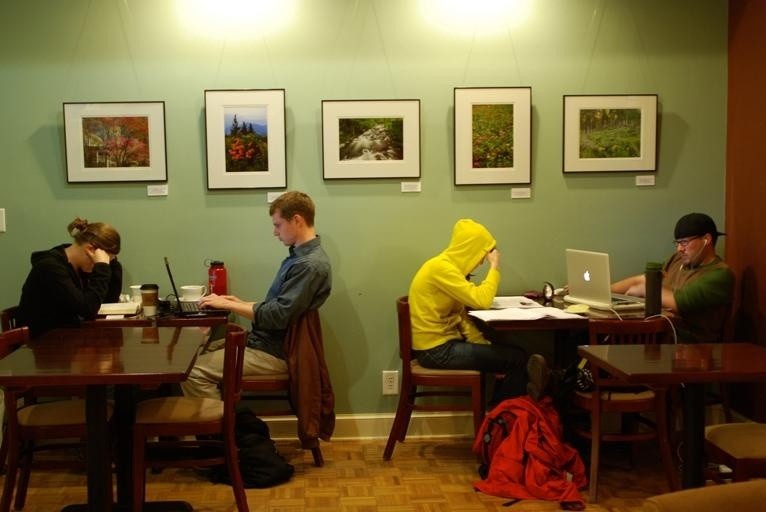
[383,369,400,396]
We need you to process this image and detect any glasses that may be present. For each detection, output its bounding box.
[673,236,699,247]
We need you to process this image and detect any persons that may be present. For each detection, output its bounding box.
[180,192,333,472]
[408,217,528,409]
[528,213,735,401]
[17,218,124,334]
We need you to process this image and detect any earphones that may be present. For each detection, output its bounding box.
[670,238,709,294]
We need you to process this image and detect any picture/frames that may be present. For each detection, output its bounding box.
[452,86,535,187]
[320,98,424,182]
[562,93,660,176]
[64,100,168,185]
[203,88,288,191]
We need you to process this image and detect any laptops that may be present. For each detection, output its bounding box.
[562,244,644,312]
[163,257,233,316]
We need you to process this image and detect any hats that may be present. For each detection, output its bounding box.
[675,212,727,239]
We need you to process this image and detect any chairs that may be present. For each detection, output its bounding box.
[0,326,116,510]
[569,311,682,505]
[130,321,248,510]
[2,303,30,328]
[640,480,762,512]
[701,420,760,477]
[381,295,484,460]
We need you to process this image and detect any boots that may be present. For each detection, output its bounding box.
[526,354,576,402]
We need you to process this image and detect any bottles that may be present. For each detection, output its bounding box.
[644,261,665,318]
[202,258,228,297]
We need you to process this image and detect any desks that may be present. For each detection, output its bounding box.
[473,283,677,464]
[457,185,578,342]
[96,297,230,328]
[2,325,211,510]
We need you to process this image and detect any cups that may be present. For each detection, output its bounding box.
[180,285,207,300]
[140,284,159,316]
[129,285,142,303]
